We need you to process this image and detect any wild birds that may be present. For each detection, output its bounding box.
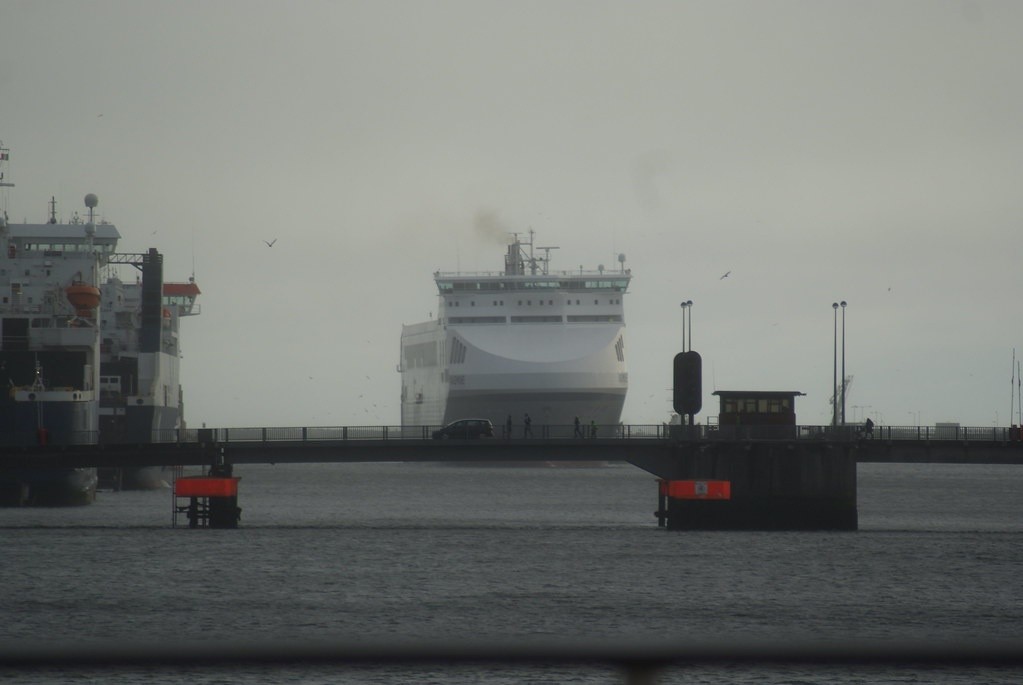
[263,238,277,248]
[719,271,731,281]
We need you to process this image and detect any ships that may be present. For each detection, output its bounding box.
[402,226,630,441]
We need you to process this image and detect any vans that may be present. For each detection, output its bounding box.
[433,419,495,441]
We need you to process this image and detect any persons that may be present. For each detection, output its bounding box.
[507,414,512,438]
[590,420,597,438]
[574,416,584,439]
[864,418,877,438]
[523,413,533,439]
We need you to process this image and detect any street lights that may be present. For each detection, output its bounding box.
[832,302,839,424]
[686,299,702,426]
[840,300,847,424]
[673,301,688,426]
